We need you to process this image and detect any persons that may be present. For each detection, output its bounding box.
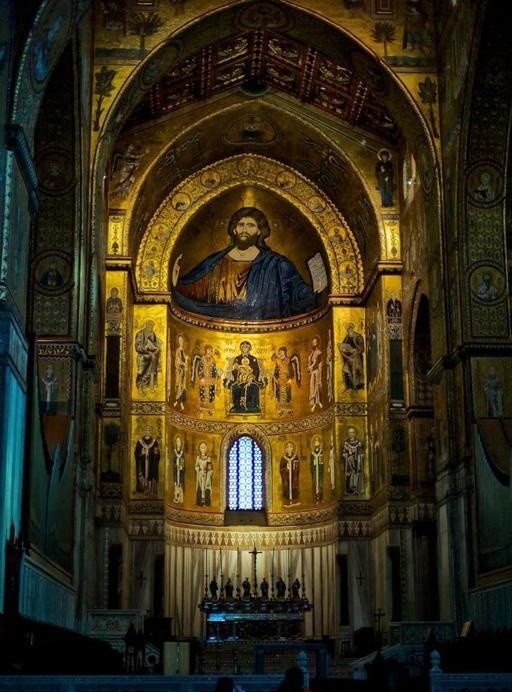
[209,575,301,599]
[273,667,306,691]
[216,677,241,692]
